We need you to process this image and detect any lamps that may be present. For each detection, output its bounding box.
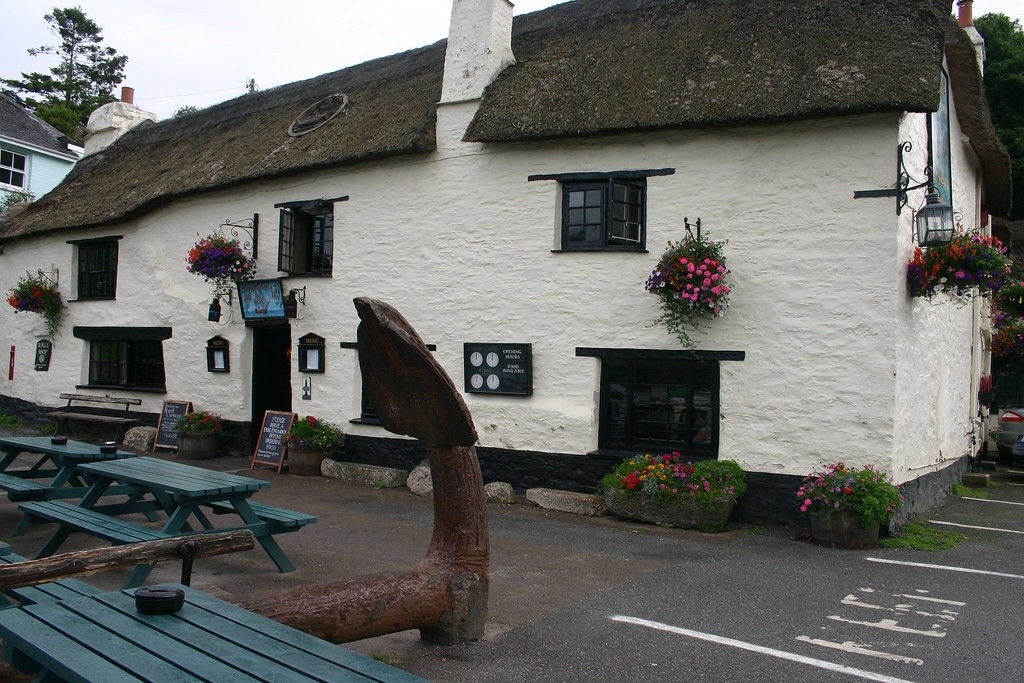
[282,284,307,319]
[896,141,963,246]
[208,289,233,322]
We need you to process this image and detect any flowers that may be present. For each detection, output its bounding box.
[788,459,908,529]
[184,228,258,289]
[907,226,1016,305]
[286,415,349,455]
[594,451,748,509]
[639,224,737,367]
[171,410,225,439]
[4,270,70,345]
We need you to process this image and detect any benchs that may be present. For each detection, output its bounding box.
[0,471,318,544]
[44,392,143,442]
[0,546,103,603]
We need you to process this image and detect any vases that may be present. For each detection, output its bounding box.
[177,430,220,458]
[602,485,733,528]
[809,507,879,544]
[287,441,335,476]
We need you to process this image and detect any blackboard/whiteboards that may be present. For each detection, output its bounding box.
[253,410,298,466]
[154,400,195,449]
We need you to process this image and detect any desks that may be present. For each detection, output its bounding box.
[0,581,439,683]
[0,436,137,486]
[32,455,297,592]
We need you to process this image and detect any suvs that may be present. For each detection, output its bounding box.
[997,407,1024,465]
